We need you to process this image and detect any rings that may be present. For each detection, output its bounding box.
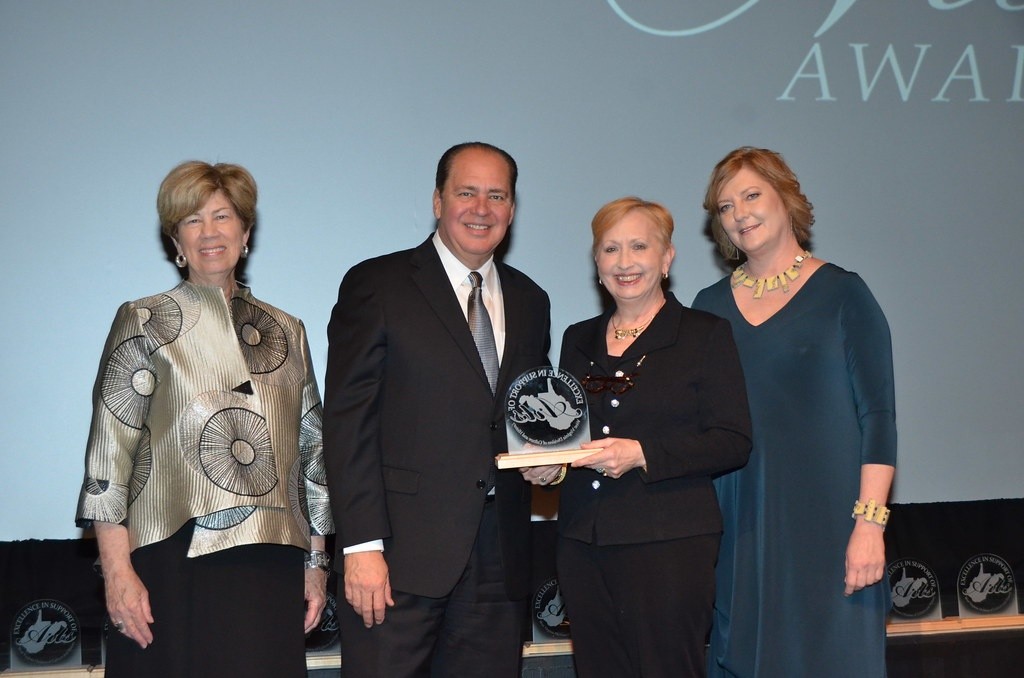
[538,476,546,482]
[113,622,124,632]
[596,467,608,476]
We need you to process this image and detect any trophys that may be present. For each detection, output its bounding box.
[494,366,605,470]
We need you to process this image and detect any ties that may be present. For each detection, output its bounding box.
[467,272,500,393]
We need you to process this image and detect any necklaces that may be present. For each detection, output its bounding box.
[612,304,653,338]
[733,250,813,298]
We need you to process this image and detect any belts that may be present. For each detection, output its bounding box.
[485,495,495,504]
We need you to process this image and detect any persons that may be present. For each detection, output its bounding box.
[321,142,552,678]
[517,197,753,678]
[690,145,898,678]
[77,162,337,678]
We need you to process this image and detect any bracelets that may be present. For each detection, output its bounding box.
[305,549,332,575]
[851,499,891,531]
